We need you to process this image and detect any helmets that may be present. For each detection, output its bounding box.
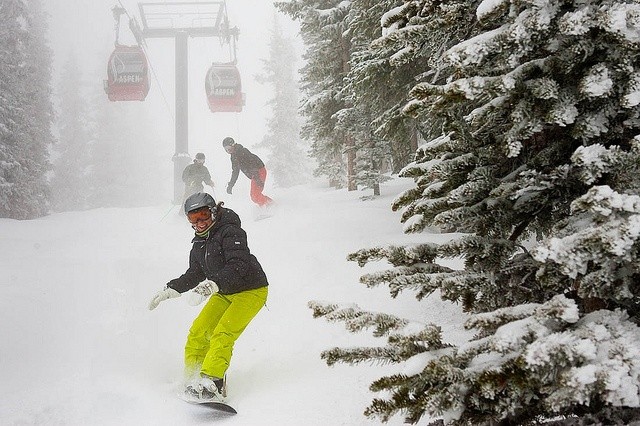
[222,137,234,153]
[184,192,217,222]
[195,153,205,161]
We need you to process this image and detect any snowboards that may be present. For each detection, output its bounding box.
[179,393,237,414]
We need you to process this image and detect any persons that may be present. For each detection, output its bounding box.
[222,137,275,209]
[178,153,216,215]
[147,192,269,401]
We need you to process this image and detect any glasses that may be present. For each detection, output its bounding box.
[187,205,211,224]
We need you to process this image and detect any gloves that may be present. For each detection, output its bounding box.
[227,186,232,194]
[255,178,260,186]
[146,287,181,311]
[187,279,220,306]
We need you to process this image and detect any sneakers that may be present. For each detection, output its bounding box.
[184,378,223,400]
